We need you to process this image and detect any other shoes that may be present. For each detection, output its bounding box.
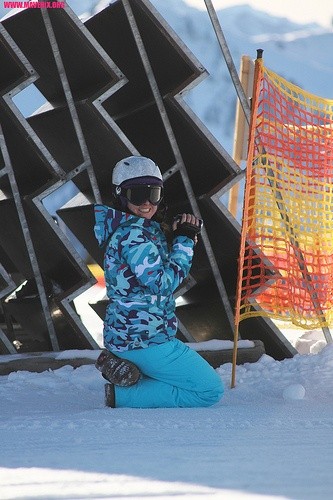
[104,383,115,409]
[94,349,141,386]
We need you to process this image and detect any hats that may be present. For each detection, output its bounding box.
[112,156,164,188]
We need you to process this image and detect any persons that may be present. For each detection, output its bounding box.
[95,156,227,411]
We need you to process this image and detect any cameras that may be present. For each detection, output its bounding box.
[173,215,204,235]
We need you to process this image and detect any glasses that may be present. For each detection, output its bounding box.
[117,185,164,206]
